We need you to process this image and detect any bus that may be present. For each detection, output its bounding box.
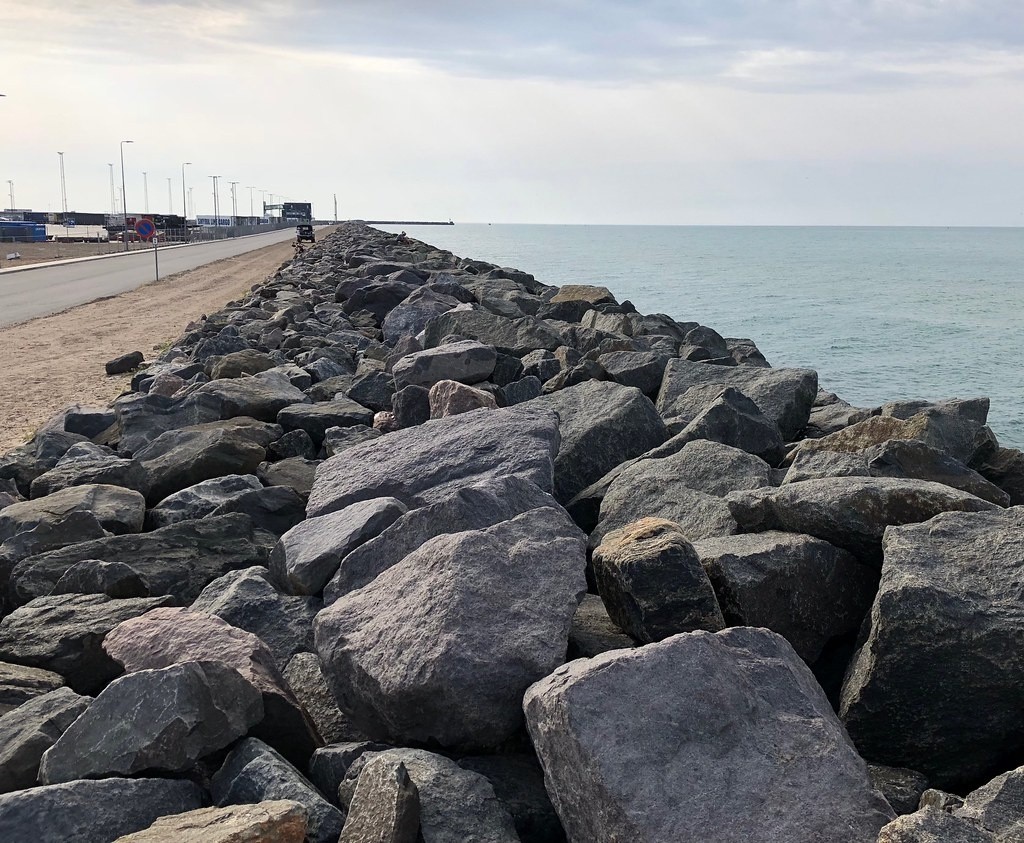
[0,221,46,243]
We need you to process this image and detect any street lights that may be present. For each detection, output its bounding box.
[228,181,239,238]
[57,151,66,224]
[277,196,282,225]
[9,179,13,216]
[120,140,134,251]
[268,193,274,228]
[143,172,148,214]
[182,162,191,242]
[115,199,119,212]
[167,178,171,213]
[108,163,115,215]
[246,187,255,231]
[210,176,222,240]
[258,189,267,226]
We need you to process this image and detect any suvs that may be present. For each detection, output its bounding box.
[296,225,316,243]
